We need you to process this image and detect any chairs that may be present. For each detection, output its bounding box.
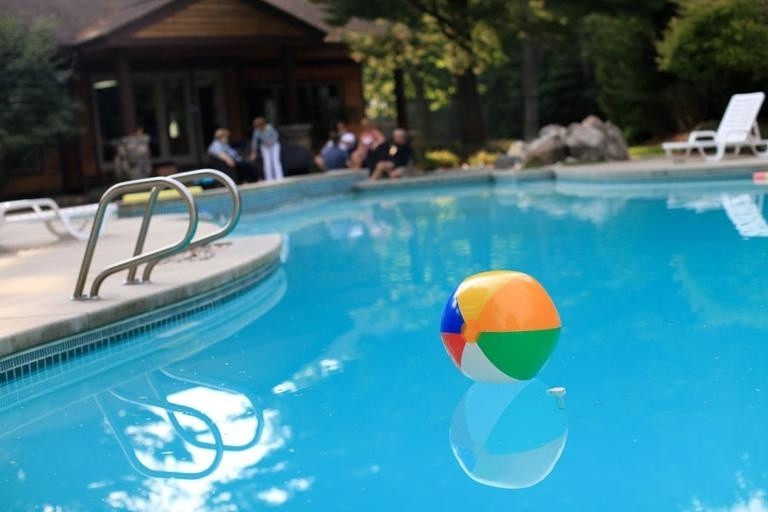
[661,92,768,163]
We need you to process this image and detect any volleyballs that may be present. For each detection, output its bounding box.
[439,270,561,385]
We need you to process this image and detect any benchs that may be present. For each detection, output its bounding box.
[1,192,118,251]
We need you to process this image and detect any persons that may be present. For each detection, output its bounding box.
[314,118,413,184]
[208,129,258,182]
[250,117,284,180]
